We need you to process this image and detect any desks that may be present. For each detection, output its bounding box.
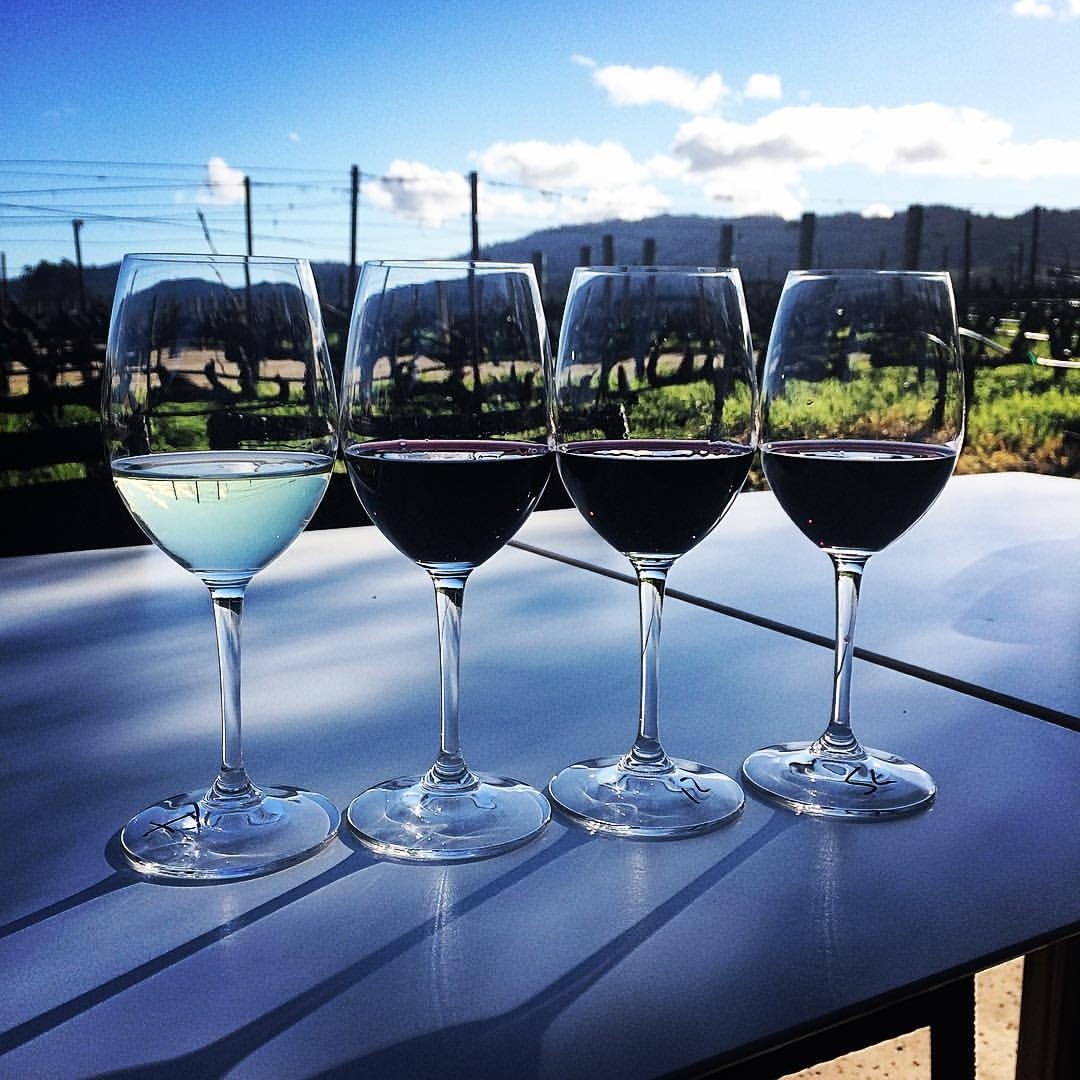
[0,472,1080,1080]
